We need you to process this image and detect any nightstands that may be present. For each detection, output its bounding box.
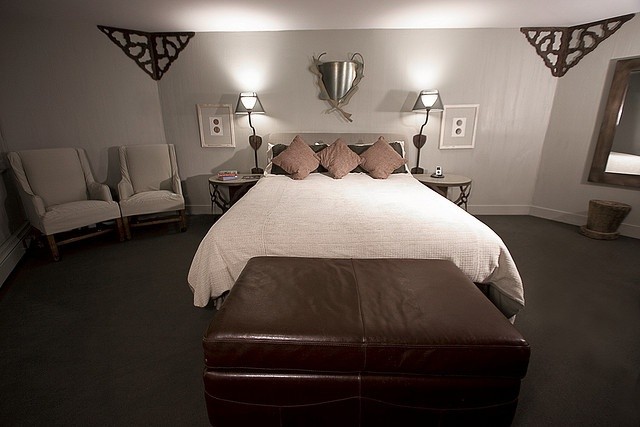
[413,174,472,212]
[209,173,262,218]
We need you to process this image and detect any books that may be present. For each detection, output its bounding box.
[217,171,238,181]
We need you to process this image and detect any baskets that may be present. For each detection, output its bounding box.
[577,198,633,241]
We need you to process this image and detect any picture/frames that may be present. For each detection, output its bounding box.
[197,104,235,148]
[439,104,480,150]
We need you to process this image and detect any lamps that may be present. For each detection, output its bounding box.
[411,89,444,174]
[235,92,266,175]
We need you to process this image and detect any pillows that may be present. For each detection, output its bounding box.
[313,138,365,179]
[359,136,408,179]
[264,142,330,178]
[347,141,409,175]
[271,135,320,179]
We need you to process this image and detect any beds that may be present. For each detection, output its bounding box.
[188,133,525,327]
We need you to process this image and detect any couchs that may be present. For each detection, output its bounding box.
[7,147,126,262]
[117,144,188,239]
[202,255,531,427]
